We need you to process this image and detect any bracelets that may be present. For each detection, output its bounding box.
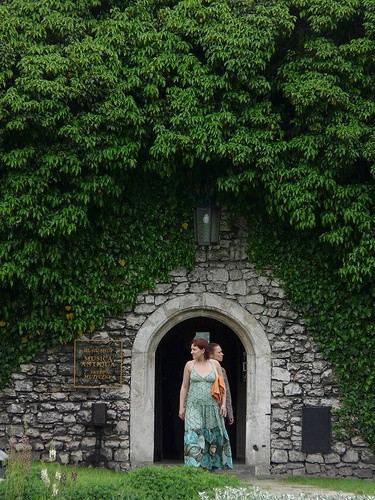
[221,404,226,407]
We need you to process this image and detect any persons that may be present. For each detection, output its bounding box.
[184,343,234,424]
[178,338,228,472]
